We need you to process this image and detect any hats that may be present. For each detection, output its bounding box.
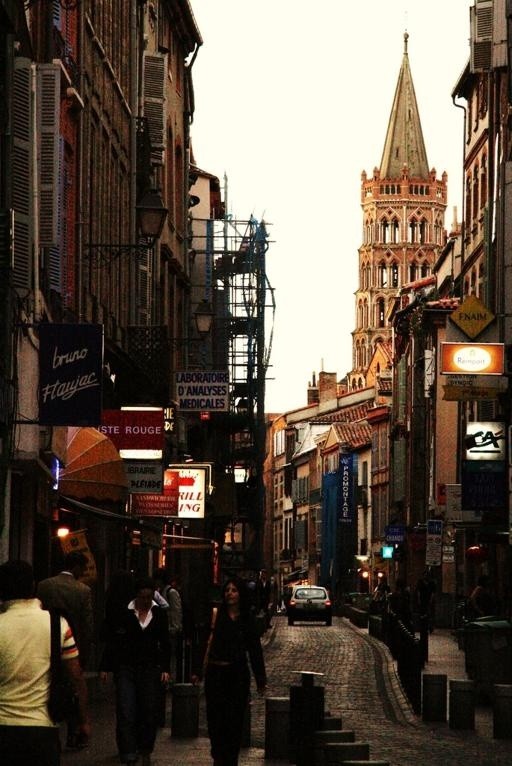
[0,560,34,600]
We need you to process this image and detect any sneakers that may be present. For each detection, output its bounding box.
[65,734,78,751]
[78,729,92,747]
[124,754,138,765]
[142,754,151,766]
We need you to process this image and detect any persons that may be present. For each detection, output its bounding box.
[0,558,92,764]
[99,565,193,766]
[450,573,488,637]
[36,550,94,751]
[187,574,267,765]
[255,566,273,629]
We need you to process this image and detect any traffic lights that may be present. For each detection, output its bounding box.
[376,571,384,578]
[361,571,368,579]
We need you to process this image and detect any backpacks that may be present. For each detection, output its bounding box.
[181,604,197,642]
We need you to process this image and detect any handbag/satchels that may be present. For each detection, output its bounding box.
[48,671,77,721]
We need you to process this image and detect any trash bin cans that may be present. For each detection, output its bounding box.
[287,670,324,764]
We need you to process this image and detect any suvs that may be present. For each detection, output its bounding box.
[286,583,333,626]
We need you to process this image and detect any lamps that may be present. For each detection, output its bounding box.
[86,185,169,267]
[52,527,70,538]
[154,299,217,350]
